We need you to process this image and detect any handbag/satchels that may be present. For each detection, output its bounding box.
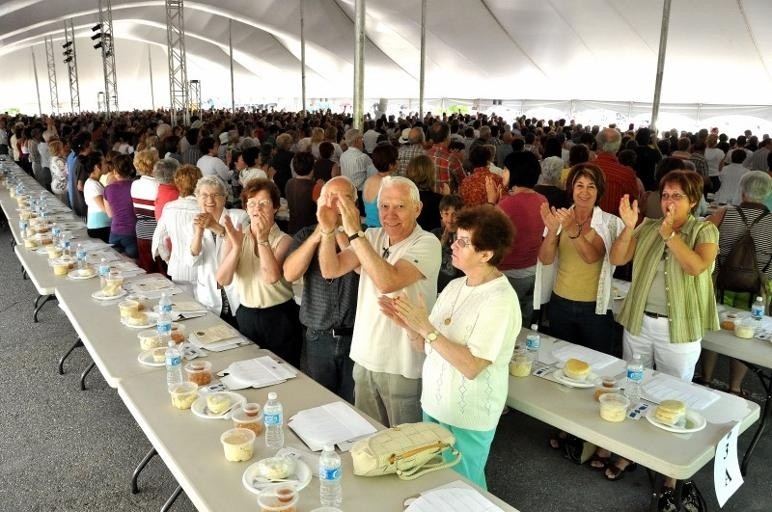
[561,436,599,465]
[649,480,707,512]
[349,422,461,480]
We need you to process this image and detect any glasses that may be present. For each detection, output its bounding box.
[246,198,272,208]
[453,234,470,248]
[662,192,687,201]
[198,192,223,199]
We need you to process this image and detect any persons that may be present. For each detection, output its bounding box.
[282,175,375,407]
[212,176,305,373]
[598,166,724,512]
[532,157,631,473]
[316,173,444,430]
[489,147,553,418]
[376,203,525,491]
[696,169,772,399]
[1,105,772,334]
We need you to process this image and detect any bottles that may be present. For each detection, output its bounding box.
[319,443,344,505]
[750,296,763,333]
[526,323,541,366]
[264,391,284,448]
[1,154,124,296]
[166,339,185,385]
[625,352,645,403]
[158,292,173,338]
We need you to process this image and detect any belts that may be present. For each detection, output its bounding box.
[644,311,668,318]
[318,327,354,338]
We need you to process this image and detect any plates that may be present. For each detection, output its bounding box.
[138,347,183,367]
[646,407,707,433]
[192,391,245,418]
[122,311,160,329]
[92,289,127,301]
[243,456,313,494]
[612,283,626,299]
[552,370,600,387]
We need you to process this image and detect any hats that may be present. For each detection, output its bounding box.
[399,128,412,144]
[218,132,229,144]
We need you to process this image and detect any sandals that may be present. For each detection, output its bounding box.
[588,453,637,481]
[549,433,566,450]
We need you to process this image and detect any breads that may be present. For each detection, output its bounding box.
[152,348,166,362]
[656,399,685,425]
[127,313,149,326]
[258,457,296,480]
[564,359,591,379]
[206,392,231,415]
[80,266,94,275]
[103,284,120,296]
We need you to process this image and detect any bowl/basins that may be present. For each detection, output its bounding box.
[138,329,160,350]
[716,311,755,340]
[171,322,185,344]
[232,402,264,436]
[119,300,139,317]
[221,428,256,462]
[593,375,621,402]
[184,359,212,386]
[168,381,198,409]
[598,392,630,422]
[509,353,533,377]
[256,482,299,512]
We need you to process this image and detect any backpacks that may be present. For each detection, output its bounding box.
[715,205,772,294]
